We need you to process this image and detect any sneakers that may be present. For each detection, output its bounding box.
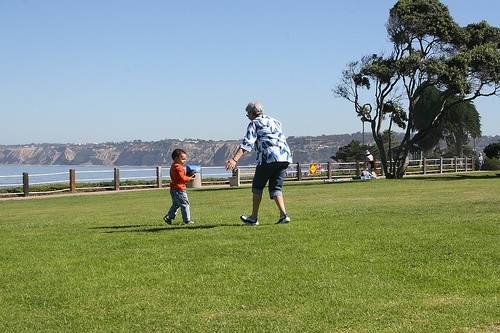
[183,221,194,225]
[240,215,259,226]
[163,216,171,224]
[276,214,290,224]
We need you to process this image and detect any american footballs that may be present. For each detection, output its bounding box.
[186,170,196,182]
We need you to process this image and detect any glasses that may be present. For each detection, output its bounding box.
[246,114,248,116]
